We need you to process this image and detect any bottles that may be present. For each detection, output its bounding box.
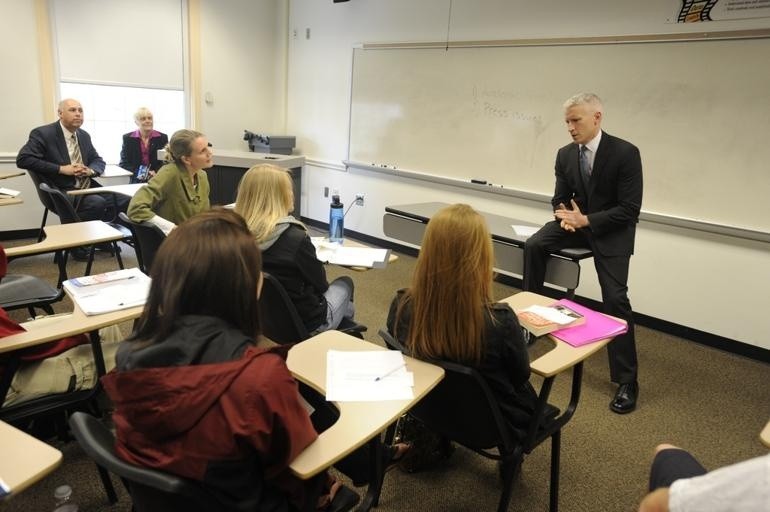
[51,483,83,512]
[329,196,344,242]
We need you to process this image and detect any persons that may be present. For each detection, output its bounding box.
[119,107,169,183]
[521,94,643,414]
[386,204,546,452]
[232,165,355,334]
[639,443,770,512]
[99,207,412,512]
[126,131,214,235]
[16,99,131,262]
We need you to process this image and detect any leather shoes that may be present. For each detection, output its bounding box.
[67,246,94,262]
[352,442,414,488]
[609,380,639,414]
[95,242,121,253]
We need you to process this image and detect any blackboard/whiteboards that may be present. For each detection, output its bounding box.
[343,28,770,243]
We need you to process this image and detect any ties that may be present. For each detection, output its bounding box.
[579,146,592,178]
[68,135,90,189]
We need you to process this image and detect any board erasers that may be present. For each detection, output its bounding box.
[470,180,486,184]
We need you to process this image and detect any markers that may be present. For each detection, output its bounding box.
[489,184,503,188]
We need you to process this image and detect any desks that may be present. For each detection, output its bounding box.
[384,201,594,290]
[285,328,444,479]
[2,420,64,507]
[496,291,629,378]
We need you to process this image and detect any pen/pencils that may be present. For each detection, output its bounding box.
[376,363,407,380]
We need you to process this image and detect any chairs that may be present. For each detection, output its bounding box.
[1,148,400,425]
[377,328,562,511]
[70,412,206,511]
